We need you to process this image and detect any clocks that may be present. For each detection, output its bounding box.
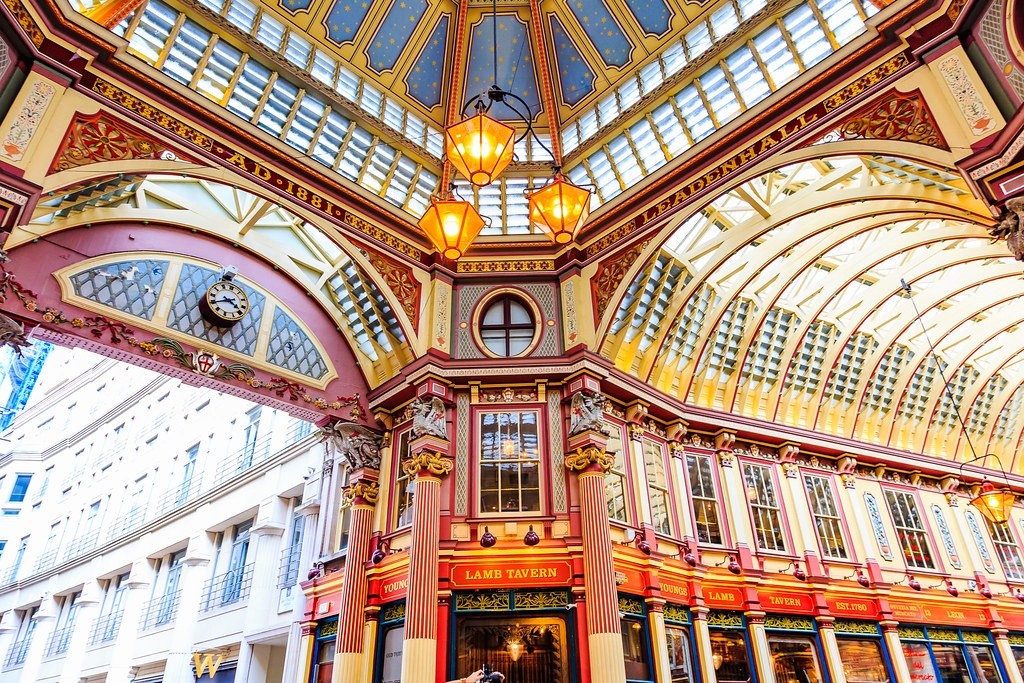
[199,279,250,328]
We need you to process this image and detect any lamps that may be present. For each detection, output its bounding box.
[843,568,869,587]
[670,546,696,568]
[931,578,959,597]
[308,561,338,580]
[372,541,402,565]
[480,526,496,547]
[901,277,1016,524]
[503,420,515,456]
[621,533,651,556]
[1005,587,1024,602]
[715,555,741,575]
[895,574,921,591]
[972,582,991,599]
[779,562,805,581]
[524,526,540,546]
[417,0,598,260]
